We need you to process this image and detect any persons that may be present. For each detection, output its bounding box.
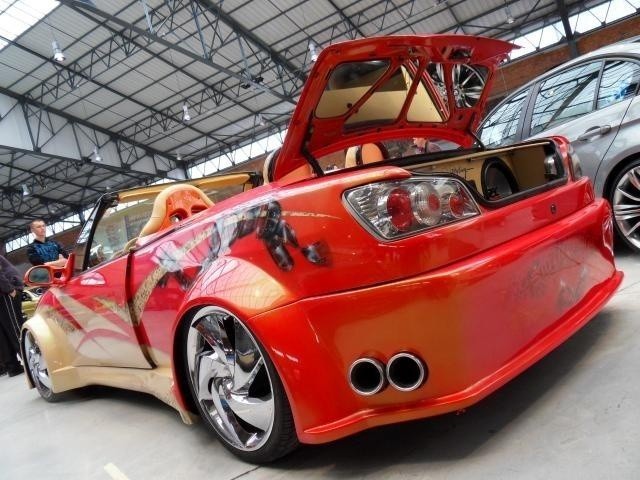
[412,136,443,154]
[27,219,70,279]
[387,148,403,160]
[0,253,28,378]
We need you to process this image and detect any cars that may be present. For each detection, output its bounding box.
[475,34,640,254]
[18,34,624,465]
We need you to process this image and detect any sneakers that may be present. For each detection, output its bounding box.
[0,360,24,377]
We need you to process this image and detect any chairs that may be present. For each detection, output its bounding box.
[137,182,216,238]
[260,140,391,187]
